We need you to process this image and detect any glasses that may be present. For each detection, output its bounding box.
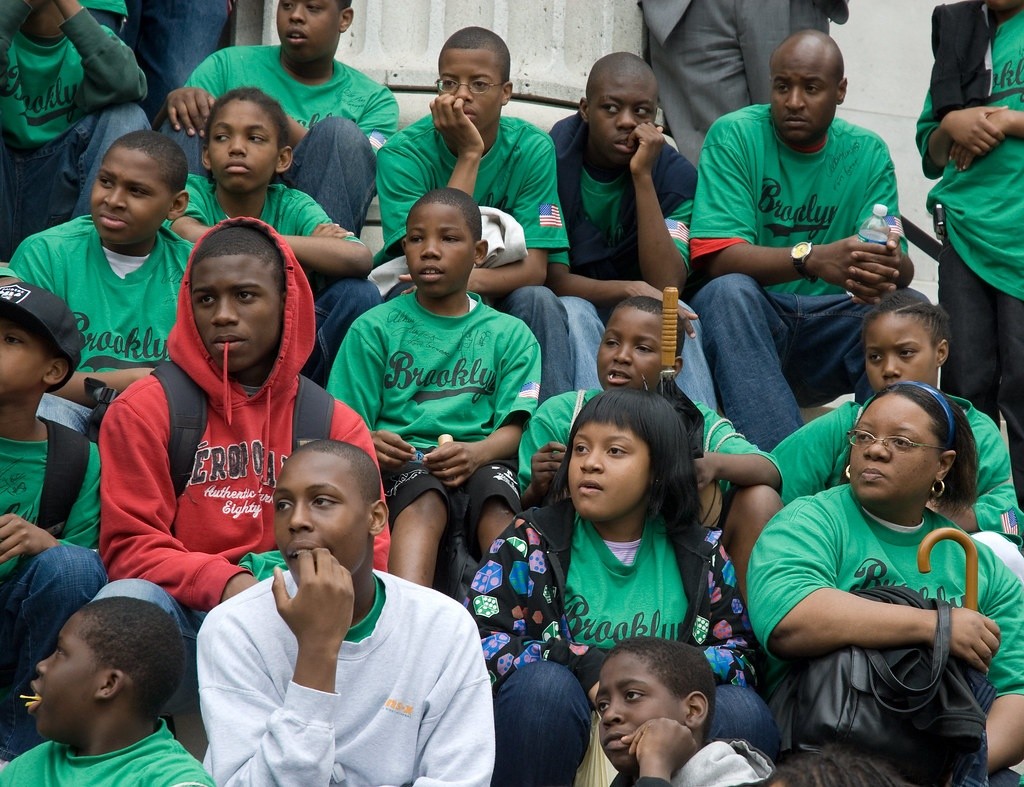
[435,78,506,94]
[846,428,948,456]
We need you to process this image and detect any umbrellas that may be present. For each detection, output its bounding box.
[917,527,999,787]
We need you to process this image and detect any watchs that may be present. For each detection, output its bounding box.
[790,242,818,284]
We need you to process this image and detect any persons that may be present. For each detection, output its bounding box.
[0,0,152,262]
[773,287,1024,548]
[328,187,542,590]
[163,87,382,367]
[0,595,217,787]
[594,638,777,787]
[152,0,400,239]
[747,380,1024,787]
[462,387,776,787]
[689,28,930,453]
[9,129,196,435]
[97,216,391,718]
[548,50,716,412]
[79,0,229,125]
[519,296,783,581]
[638,0,850,173]
[195,439,496,787]
[915,0,1024,508]
[376,26,577,406]
[0,281,109,763]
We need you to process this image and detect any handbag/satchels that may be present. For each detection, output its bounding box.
[791,585,957,787]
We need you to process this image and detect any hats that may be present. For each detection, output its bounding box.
[0,284,82,392]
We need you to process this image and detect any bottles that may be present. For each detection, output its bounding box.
[846,203,889,298]
